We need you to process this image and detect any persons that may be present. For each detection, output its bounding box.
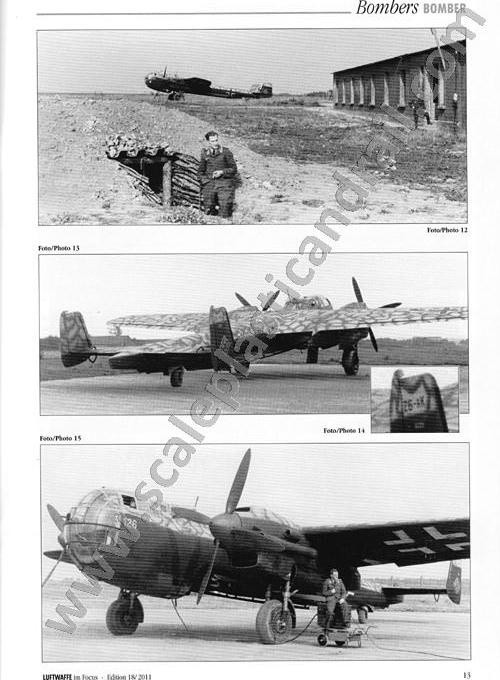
[197,130,238,216]
[325,567,350,628]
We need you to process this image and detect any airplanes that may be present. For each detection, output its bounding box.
[57,275,469,388]
[144,65,273,103]
[42,445,470,646]
